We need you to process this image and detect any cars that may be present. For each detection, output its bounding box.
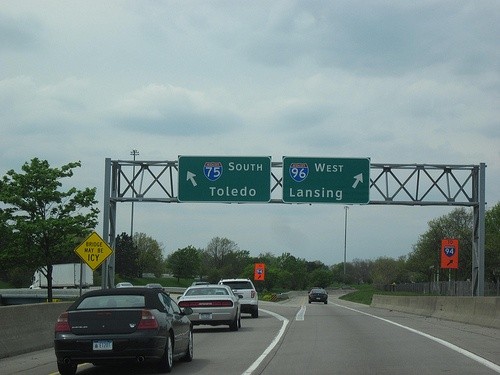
[115,282,134,289]
[176,283,244,332]
[53,286,194,375]
[145,282,165,291]
[307,287,329,304]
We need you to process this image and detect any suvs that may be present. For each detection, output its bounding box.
[213,276,259,318]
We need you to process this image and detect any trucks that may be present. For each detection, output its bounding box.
[29,262,94,289]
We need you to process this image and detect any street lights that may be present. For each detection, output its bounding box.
[343,206,349,276]
[129,149,140,237]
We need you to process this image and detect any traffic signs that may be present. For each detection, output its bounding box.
[282,155,371,206]
[177,154,272,204]
